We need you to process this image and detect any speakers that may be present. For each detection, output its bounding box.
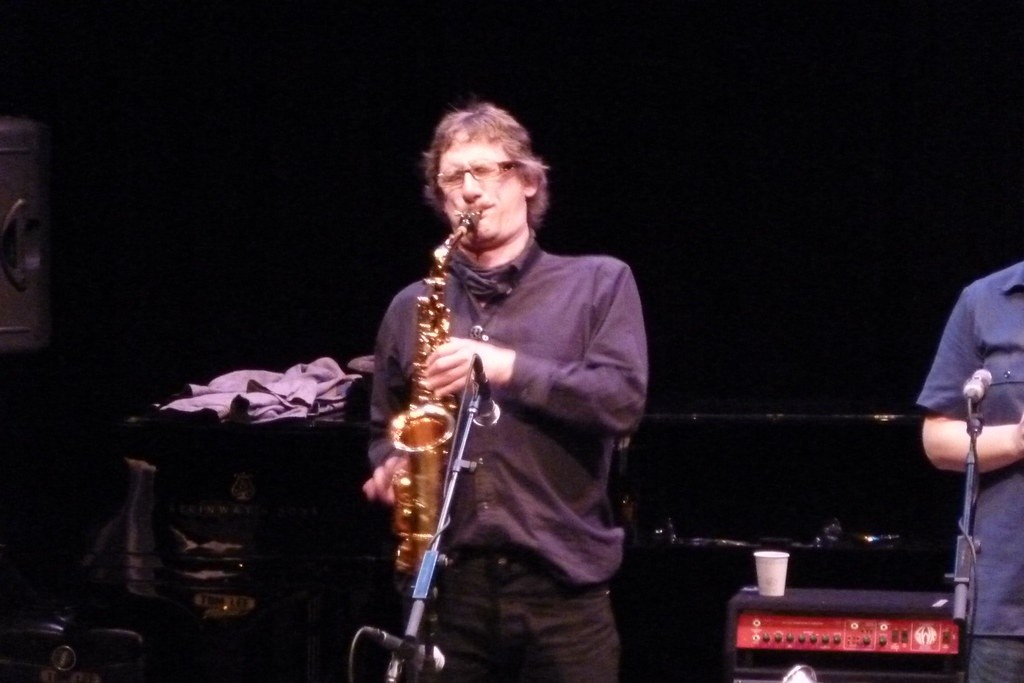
[0,116,50,350]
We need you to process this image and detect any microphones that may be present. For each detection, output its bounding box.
[363,626,445,673]
[468,354,501,426]
[962,370,992,403]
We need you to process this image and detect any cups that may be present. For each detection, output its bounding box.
[754,551,789,597]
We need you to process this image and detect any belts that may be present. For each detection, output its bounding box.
[438,545,531,564]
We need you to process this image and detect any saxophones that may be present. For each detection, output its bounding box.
[388,205,487,578]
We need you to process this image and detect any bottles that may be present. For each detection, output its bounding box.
[616,495,637,546]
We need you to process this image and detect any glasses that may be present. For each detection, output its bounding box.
[434,160,521,187]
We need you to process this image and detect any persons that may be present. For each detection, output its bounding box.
[917,259,1024,683]
[362,102,649,683]
